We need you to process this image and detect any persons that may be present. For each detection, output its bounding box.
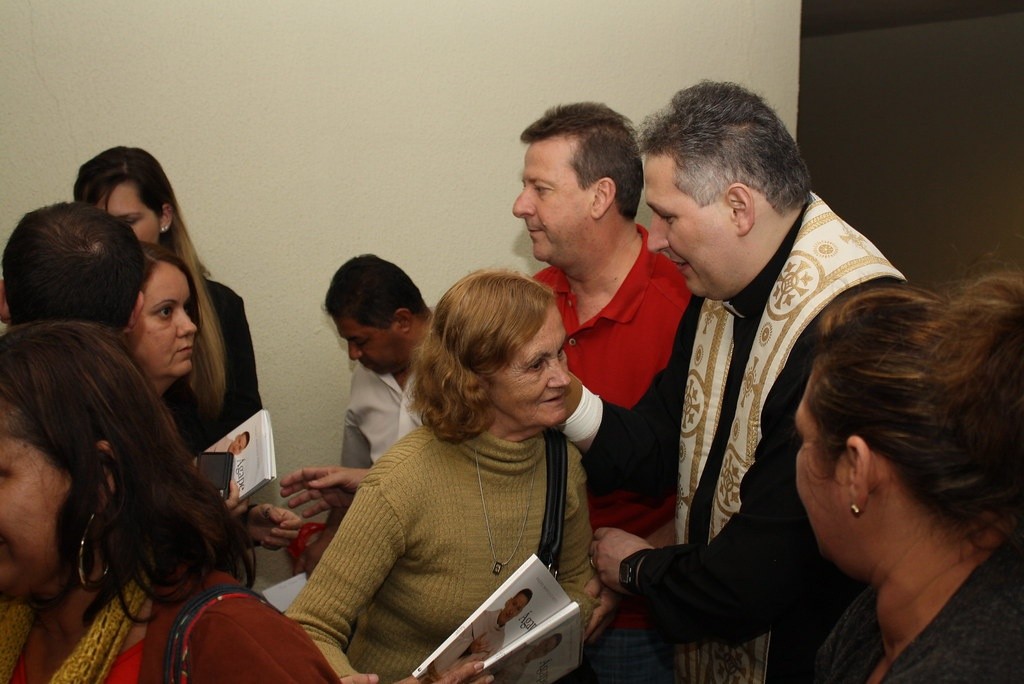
[0,324,343,684]
[1,146,302,549]
[553,81,908,684]
[793,272,1024,684]
[205,431,250,479]
[513,101,692,684]
[428,588,532,681]
[291,252,433,574]
[492,632,562,684]
[278,272,601,684]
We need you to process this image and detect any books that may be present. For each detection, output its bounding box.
[192,409,278,501]
[411,553,584,684]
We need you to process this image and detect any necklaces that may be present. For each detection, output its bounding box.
[473,442,536,576]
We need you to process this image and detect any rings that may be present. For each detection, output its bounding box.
[590,556,595,568]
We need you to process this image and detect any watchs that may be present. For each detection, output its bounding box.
[620,548,654,596]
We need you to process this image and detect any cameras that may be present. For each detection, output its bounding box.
[197,450,234,501]
